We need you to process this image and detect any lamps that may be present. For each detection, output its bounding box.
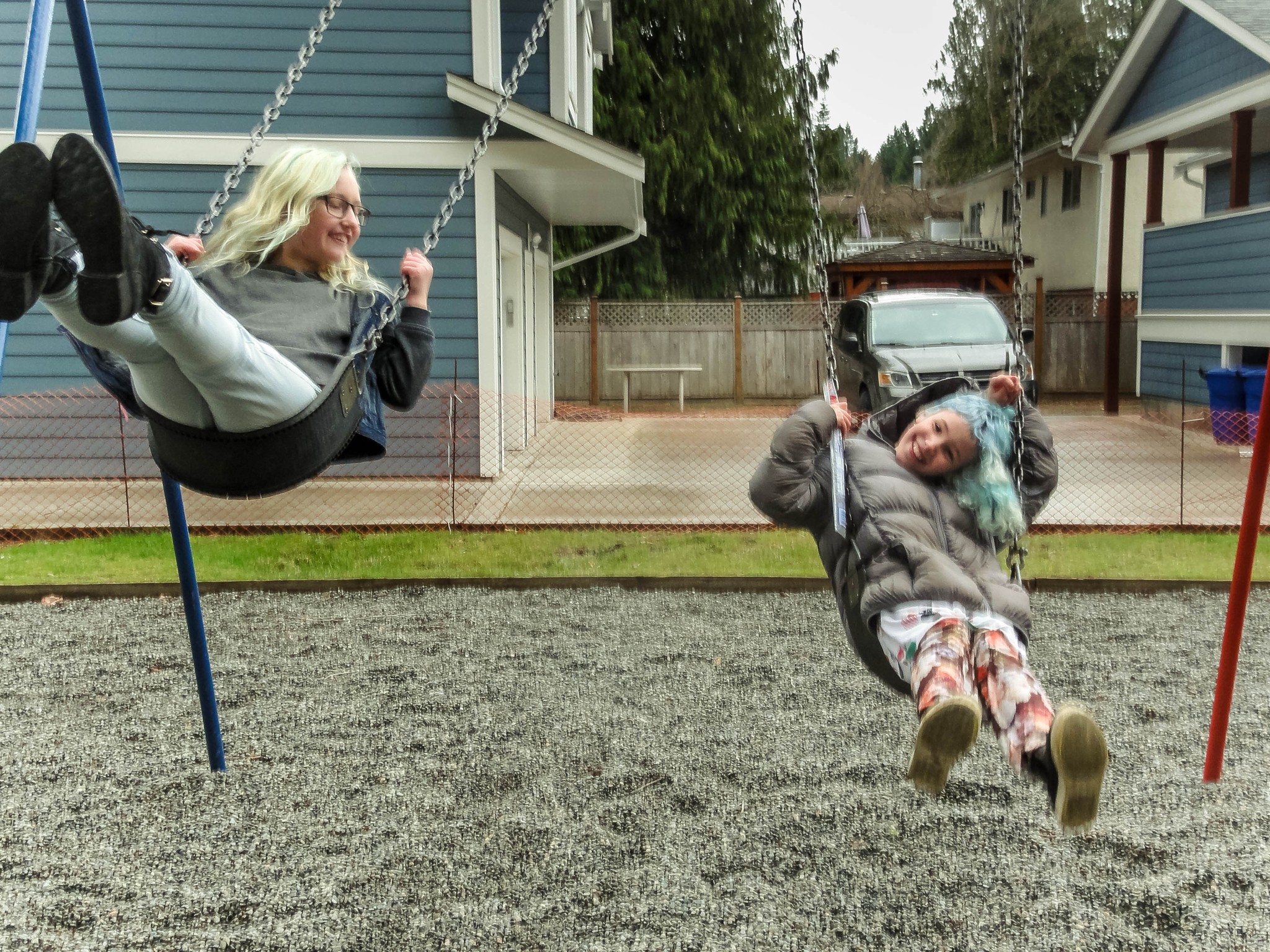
[530,232,542,250]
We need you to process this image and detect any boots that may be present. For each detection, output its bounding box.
[0,141,77,323]
[50,133,173,327]
[908,697,981,794]
[1030,706,1108,834]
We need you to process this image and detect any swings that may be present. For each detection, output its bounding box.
[131,0,551,505]
[792,0,1038,693]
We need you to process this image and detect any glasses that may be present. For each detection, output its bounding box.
[316,195,372,227]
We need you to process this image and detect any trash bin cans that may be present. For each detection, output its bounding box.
[1197,365,1268,448]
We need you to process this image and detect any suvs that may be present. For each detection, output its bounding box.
[834,287,1038,440]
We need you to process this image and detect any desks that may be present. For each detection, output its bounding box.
[606,365,702,413]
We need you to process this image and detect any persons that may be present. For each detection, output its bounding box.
[748,373,1106,827]
[0,133,434,476]
